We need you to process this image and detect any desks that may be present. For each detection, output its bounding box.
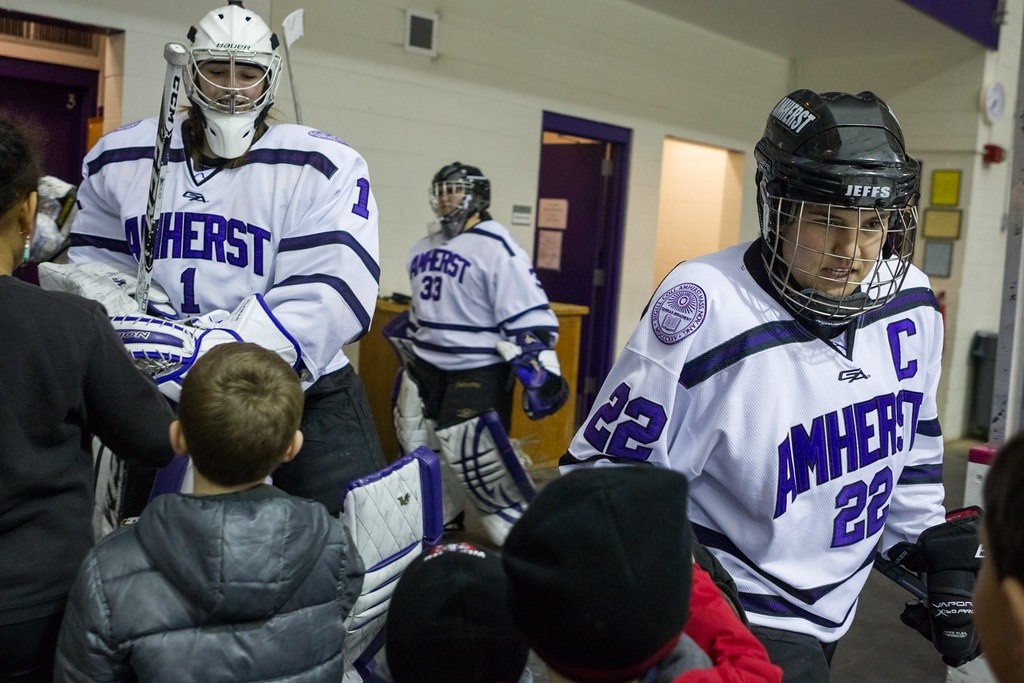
[359,296,591,469]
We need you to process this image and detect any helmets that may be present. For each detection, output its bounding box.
[432,161,490,239]
[752,87,920,328]
[187,6,281,161]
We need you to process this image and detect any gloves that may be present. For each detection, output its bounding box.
[890,505,985,670]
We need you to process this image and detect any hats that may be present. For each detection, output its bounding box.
[384,541,527,683]
[500,467,693,683]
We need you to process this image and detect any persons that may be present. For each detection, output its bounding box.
[559,89,984,683]
[0,5,783,683]
[972,428,1024,682]
